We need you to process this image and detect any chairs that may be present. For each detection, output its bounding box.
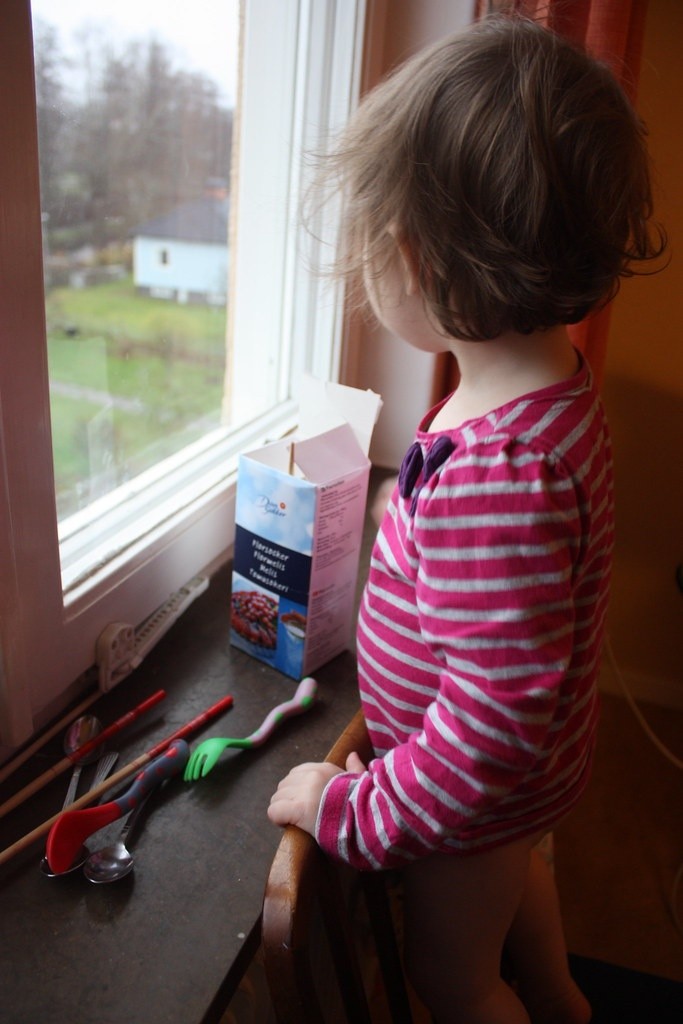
[258,704,418,1024]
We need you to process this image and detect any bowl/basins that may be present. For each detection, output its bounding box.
[283,623,305,644]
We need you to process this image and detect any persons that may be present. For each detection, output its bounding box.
[265,12,666,1024]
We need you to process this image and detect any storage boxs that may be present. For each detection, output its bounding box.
[229,381,384,683]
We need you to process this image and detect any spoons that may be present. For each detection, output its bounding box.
[47,739,189,872]
[63,715,103,808]
[45,752,119,876]
[85,795,151,883]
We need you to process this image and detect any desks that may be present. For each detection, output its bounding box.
[0,468,398,1024]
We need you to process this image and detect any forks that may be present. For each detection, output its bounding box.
[185,678,318,781]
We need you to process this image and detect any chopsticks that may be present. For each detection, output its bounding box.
[0,691,234,862]
